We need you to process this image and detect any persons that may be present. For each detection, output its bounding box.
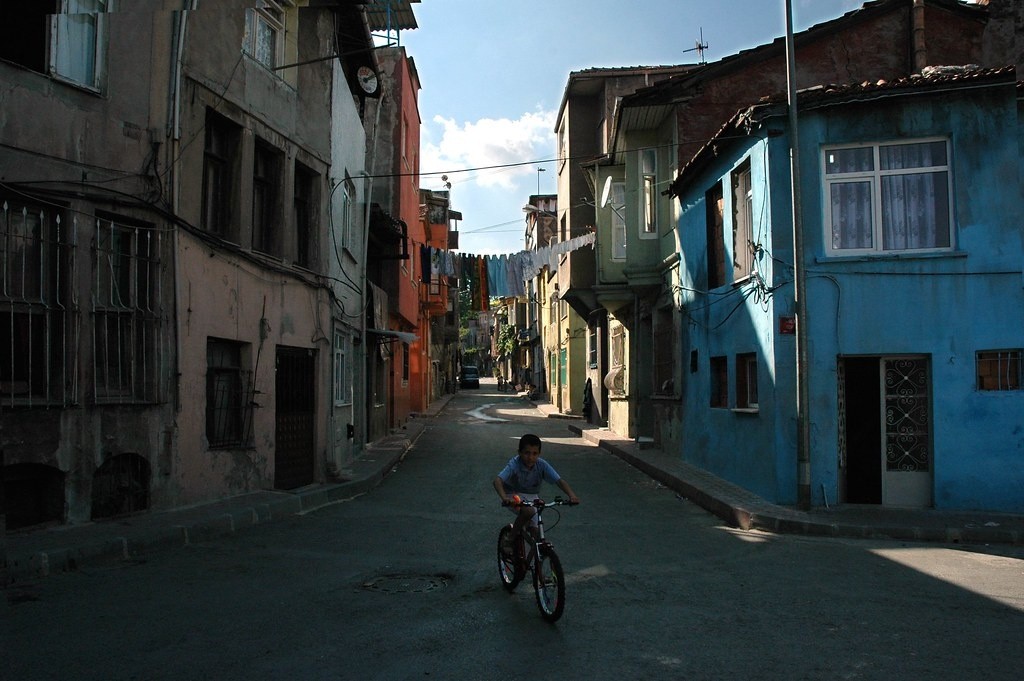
[492,434,580,584]
[497,373,503,391]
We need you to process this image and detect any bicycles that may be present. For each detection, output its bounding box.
[496,499,579,625]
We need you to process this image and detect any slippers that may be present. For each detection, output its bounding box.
[502,533,515,556]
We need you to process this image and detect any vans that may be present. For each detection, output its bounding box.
[460,365,480,390]
